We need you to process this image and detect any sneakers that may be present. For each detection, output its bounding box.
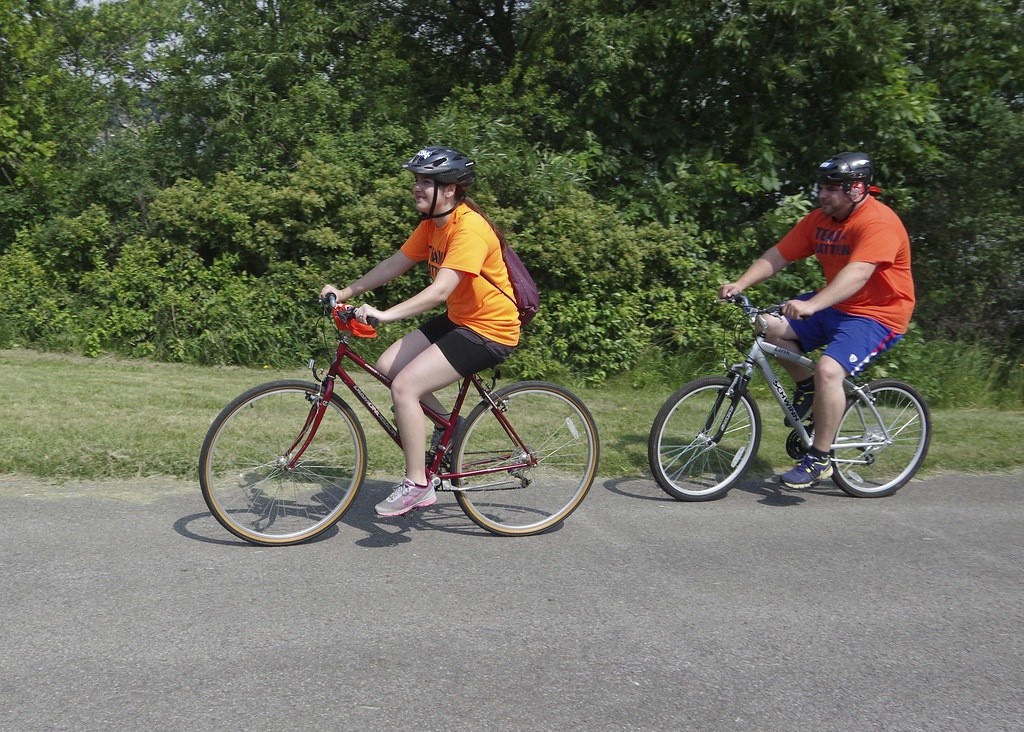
[779,453,834,488]
[374,478,437,516]
[783,382,816,428]
[431,415,467,455]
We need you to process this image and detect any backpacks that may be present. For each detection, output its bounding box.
[482,226,541,328]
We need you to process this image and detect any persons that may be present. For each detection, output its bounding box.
[719,152,916,489]
[320,146,521,518]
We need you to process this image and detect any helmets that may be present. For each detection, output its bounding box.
[402,145,477,185]
[816,150,874,188]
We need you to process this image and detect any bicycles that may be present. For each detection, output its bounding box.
[199,290,598,546]
[648,292,934,504]
